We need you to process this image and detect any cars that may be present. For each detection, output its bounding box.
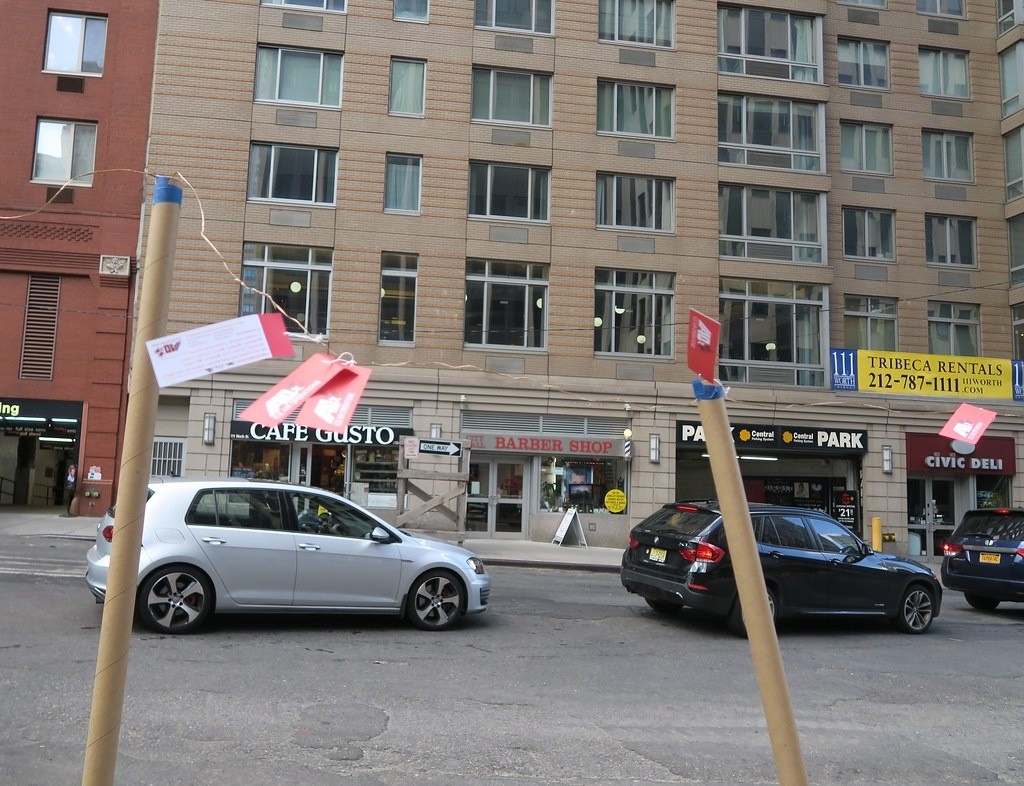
[940,494,1024,619]
[85,477,491,633]
[618,497,946,637]
